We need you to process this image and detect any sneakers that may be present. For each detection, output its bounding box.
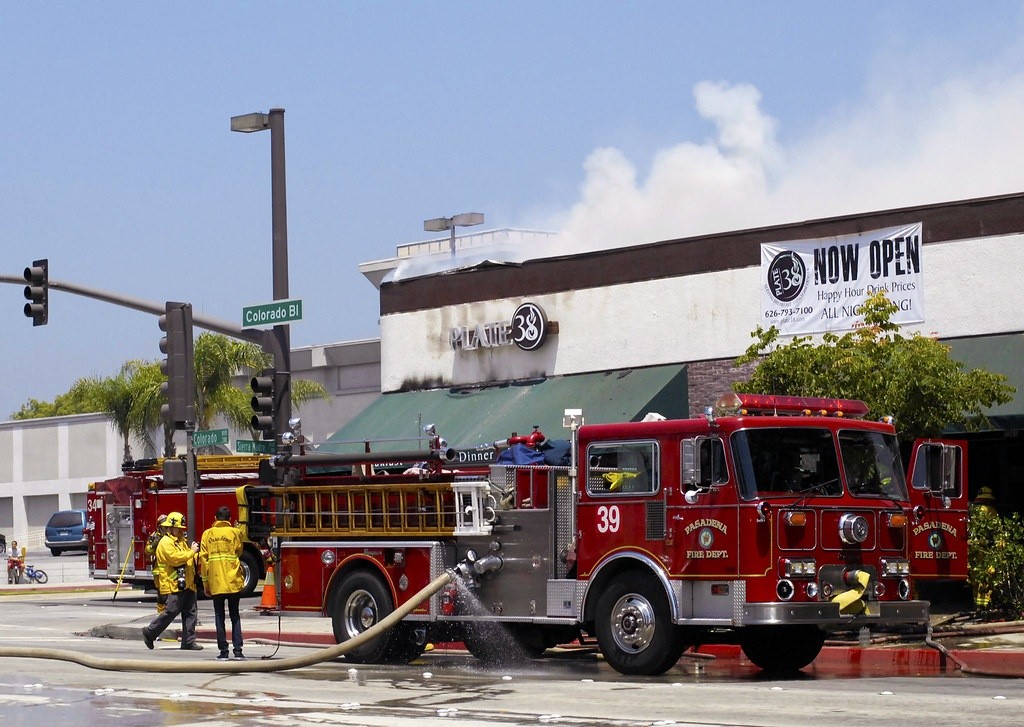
[181,642,203,650]
[142,628,154,649]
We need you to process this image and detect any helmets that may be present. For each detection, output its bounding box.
[161,512,186,528]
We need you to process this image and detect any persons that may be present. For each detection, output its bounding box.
[7,540,25,578]
[974,485,997,608]
[142,511,204,650]
[199,506,245,661]
[858,443,893,487]
[144,513,168,615]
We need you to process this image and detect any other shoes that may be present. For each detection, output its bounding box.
[216,653,229,660]
[234,652,248,660]
[9,580,12,584]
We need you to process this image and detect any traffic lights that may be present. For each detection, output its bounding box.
[249,368,278,433]
[22,257,50,327]
[157,301,195,422]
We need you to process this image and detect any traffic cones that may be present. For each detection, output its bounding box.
[253,567,278,609]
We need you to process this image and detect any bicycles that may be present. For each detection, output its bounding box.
[4,555,48,585]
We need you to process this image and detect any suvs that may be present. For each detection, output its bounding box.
[43,509,88,557]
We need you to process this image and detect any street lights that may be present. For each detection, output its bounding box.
[423,212,484,255]
[229,108,292,449]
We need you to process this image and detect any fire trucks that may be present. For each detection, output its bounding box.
[85,451,279,595]
[239,394,970,676]
